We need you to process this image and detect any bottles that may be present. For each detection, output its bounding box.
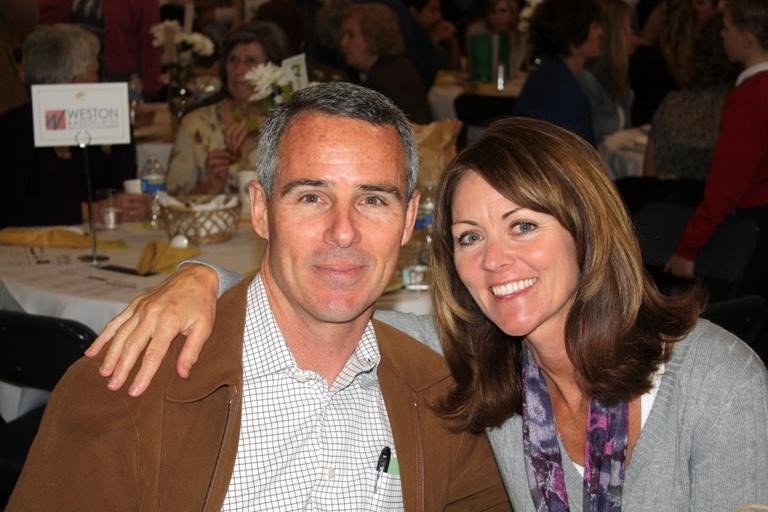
[413,181,437,266]
[128,72,143,127]
[140,154,167,232]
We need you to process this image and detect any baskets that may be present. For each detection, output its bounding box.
[166,194,243,247]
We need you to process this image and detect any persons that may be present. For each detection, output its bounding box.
[166,19,292,195]
[627,1,678,127]
[695,1,719,29]
[0,22,139,226]
[84,116,768,511]
[338,3,433,124]
[516,0,603,149]
[663,1,768,360]
[594,0,635,130]
[4,82,512,512]
[199,7,237,26]
[658,4,700,73]
[398,1,460,90]
[466,1,519,63]
[38,0,162,102]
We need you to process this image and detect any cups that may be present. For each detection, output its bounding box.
[98,188,126,230]
